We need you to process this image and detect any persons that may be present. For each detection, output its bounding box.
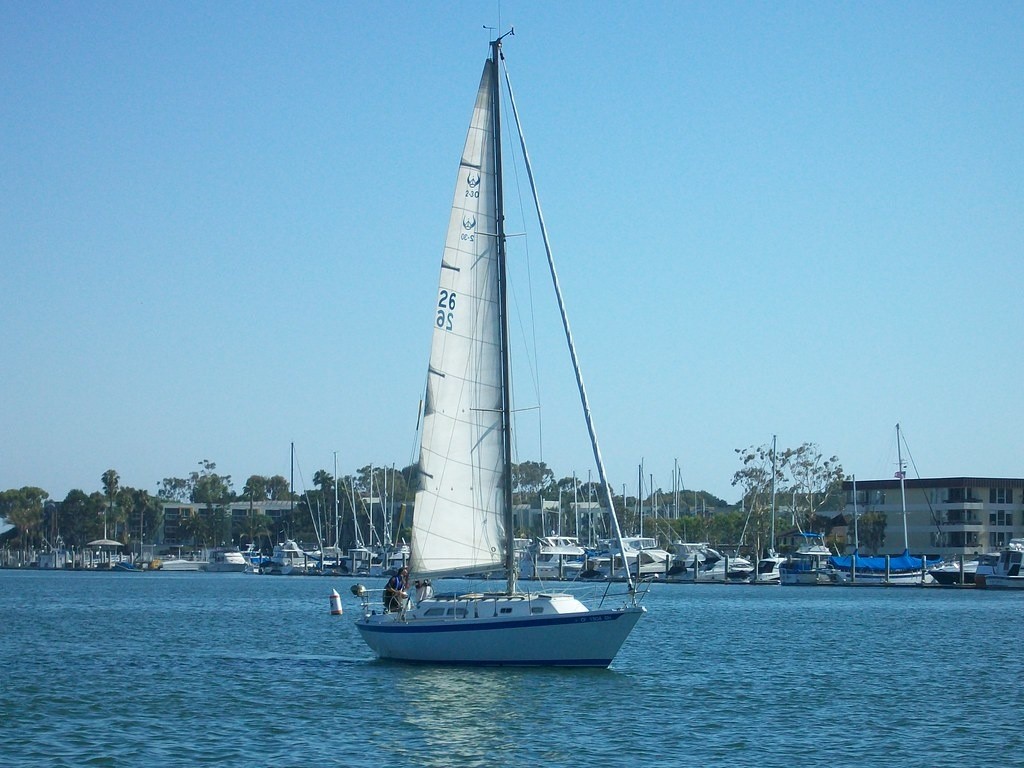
[785,553,793,569]
[383,568,408,612]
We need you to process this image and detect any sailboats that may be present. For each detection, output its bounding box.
[353,23,653,669]
[159,422,1024,592]
